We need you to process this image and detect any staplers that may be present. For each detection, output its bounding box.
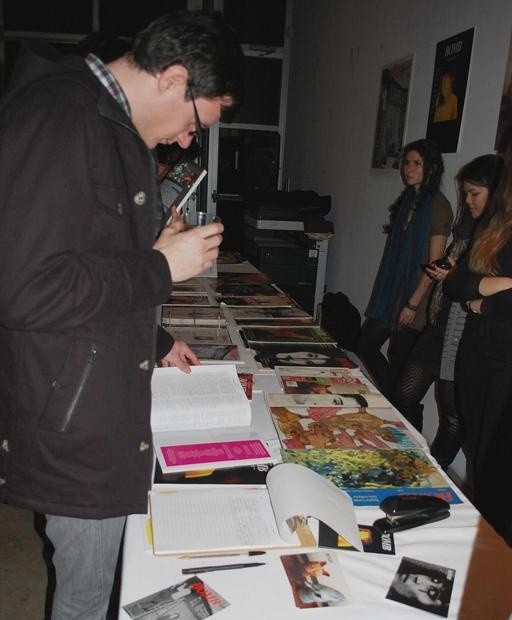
[373,494,452,535]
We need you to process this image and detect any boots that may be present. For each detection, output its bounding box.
[431,427,460,471]
[401,404,423,434]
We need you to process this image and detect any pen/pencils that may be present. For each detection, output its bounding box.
[179,552,265,559]
[182,563,267,574]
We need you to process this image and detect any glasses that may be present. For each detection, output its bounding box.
[189,86,209,147]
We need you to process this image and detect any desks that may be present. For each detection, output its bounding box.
[113,255,512,618]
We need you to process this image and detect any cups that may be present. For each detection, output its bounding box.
[176,212,221,274]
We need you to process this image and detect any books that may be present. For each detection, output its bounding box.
[159,159,207,225]
[246,215,305,233]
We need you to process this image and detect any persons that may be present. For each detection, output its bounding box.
[392,559,451,617]
[0,8,242,620]
